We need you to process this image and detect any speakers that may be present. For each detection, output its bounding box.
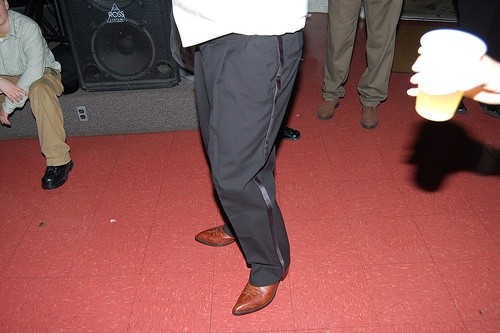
[59,0,181,91]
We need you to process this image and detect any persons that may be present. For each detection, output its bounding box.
[318,0,405,128]
[407,0,500,119]
[0,0,74,190]
[169,0,309,316]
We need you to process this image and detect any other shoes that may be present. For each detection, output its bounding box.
[478,102,500,118]
[457,97,466,114]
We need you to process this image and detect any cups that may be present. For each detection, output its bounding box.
[415,29,487,121]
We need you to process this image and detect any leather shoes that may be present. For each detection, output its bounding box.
[232,266,290,316]
[41,160,73,189]
[318,99,339,120]
[360,105,378,128]
[195,225,237,247]
